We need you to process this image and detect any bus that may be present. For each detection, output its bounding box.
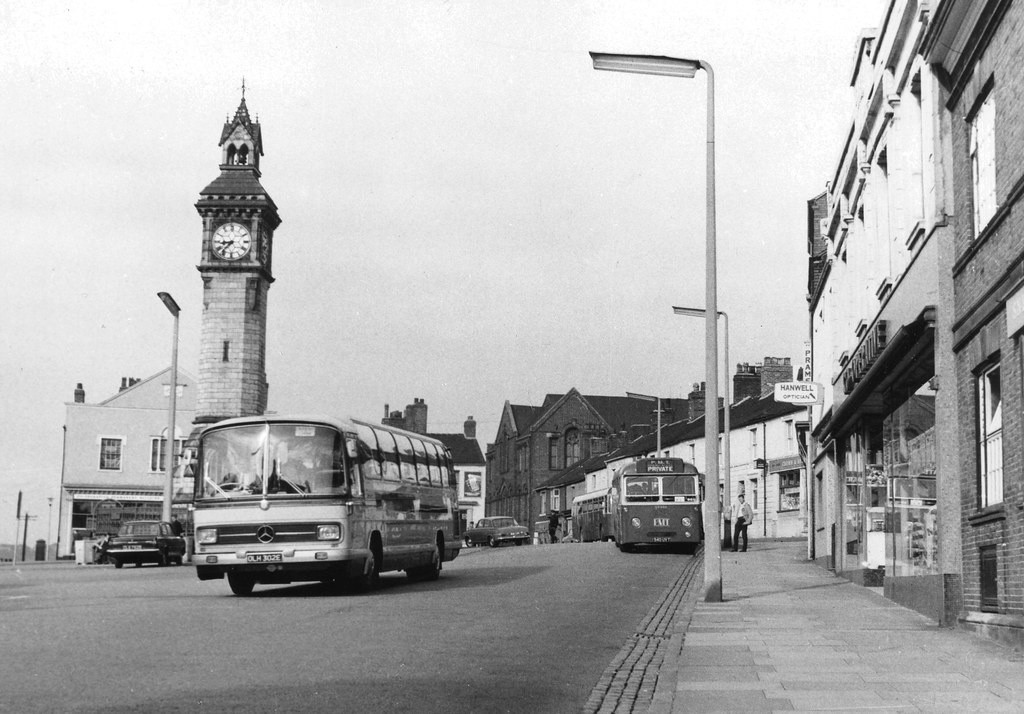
[611,458,704,554]
[572,487,616,542]
[191,414,463,596]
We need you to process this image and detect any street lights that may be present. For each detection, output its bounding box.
[46,496,54,562]
[625,392,661,458]
[673,306,735,551]
[157,291,182,524]
[587,52,722,603]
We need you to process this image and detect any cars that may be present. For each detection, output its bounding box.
[106,521,186,566]
[462,516,531,547]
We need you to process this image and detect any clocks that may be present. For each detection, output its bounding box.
[212,222,253,261]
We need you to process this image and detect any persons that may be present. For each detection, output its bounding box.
[549,510,558,544]
[94,534,110,564]
[731,494,753,553]
[170,514,184,537]
